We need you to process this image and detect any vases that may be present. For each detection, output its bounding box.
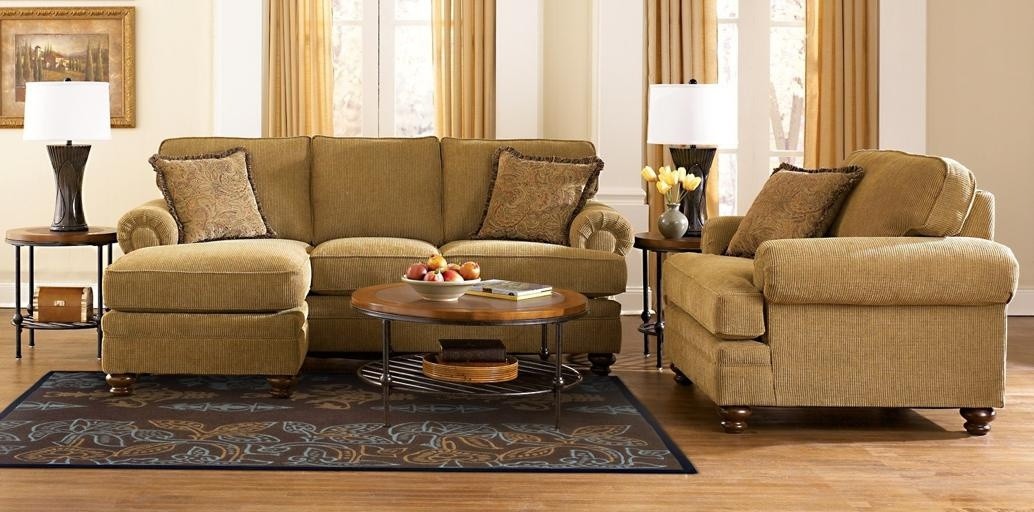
[658,203,689,239]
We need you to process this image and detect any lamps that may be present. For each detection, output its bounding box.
[23,78,112,232]
[647,79,739,237]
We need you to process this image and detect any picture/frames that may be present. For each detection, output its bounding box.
[0,7,136,128]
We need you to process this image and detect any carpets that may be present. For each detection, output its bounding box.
[1,370,698,474]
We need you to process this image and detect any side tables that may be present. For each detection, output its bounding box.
[633,232,701,370]
[6,228,118,362]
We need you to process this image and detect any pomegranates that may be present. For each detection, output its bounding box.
[406,253,481,282]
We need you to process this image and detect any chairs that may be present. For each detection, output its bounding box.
[662,149,1019,434]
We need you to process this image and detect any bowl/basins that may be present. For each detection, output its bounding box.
[400,274,481,302]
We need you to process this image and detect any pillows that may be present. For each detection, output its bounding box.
[159,137,312,244]
[312,135,443,247]
[149,146,278,244]
[724,161,864,258]
[440,137,596,244]
[829,151,976,236]
[470,146,603,246]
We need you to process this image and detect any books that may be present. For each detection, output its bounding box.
[471,286,551,302]
[438,339,505,362]
[467,275,551,294]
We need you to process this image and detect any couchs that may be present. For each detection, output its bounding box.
[101,136,635,399]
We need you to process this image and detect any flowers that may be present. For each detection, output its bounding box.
[641,165,701,204]
